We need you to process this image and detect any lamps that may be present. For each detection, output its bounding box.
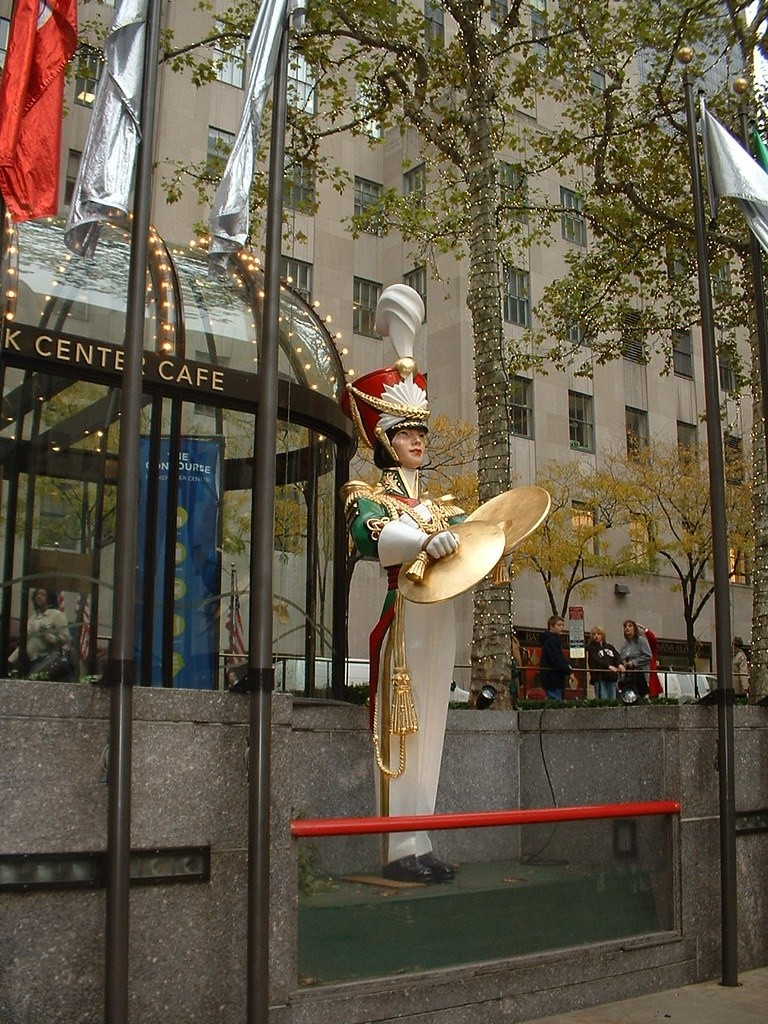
[476,684,497,710]
[621,686,640,705]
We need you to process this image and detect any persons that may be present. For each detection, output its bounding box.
[342,357,471,882]
[541,615,572,700]
[8,585,70,669]
[732,636,749,697]
[511,634,523,710]
[588,626,625,700]
[620,620,663,699]
[226,673,240,688]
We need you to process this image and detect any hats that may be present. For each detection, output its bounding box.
[733,636,743,646]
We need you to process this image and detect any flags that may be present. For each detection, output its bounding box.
[56,591,65,613]
[698,90,768,253]
[748,120,768,176]
[75,593,90,663]
[64,1,147,258]
[206,0,306,275]
[0,0,78,224]
[225,569,246,675]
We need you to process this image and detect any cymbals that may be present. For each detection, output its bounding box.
[396,519,507,606]
[464,483,553,556]
